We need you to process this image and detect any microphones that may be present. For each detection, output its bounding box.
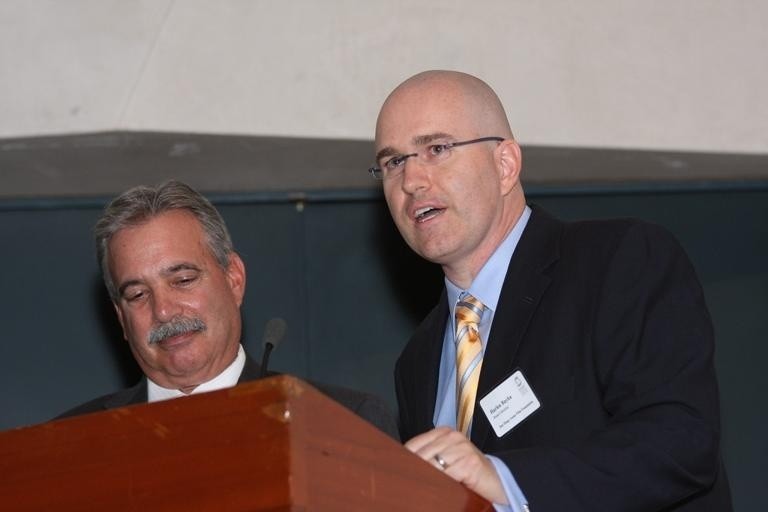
[261,318,286,377]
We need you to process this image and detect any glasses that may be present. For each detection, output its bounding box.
[367,136,508,181]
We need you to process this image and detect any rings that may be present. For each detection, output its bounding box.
[434,454,448,470]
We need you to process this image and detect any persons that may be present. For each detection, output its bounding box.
[51,179,403,445]
[368,69,733,511]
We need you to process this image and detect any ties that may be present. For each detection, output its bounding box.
[451,292,487,442]
